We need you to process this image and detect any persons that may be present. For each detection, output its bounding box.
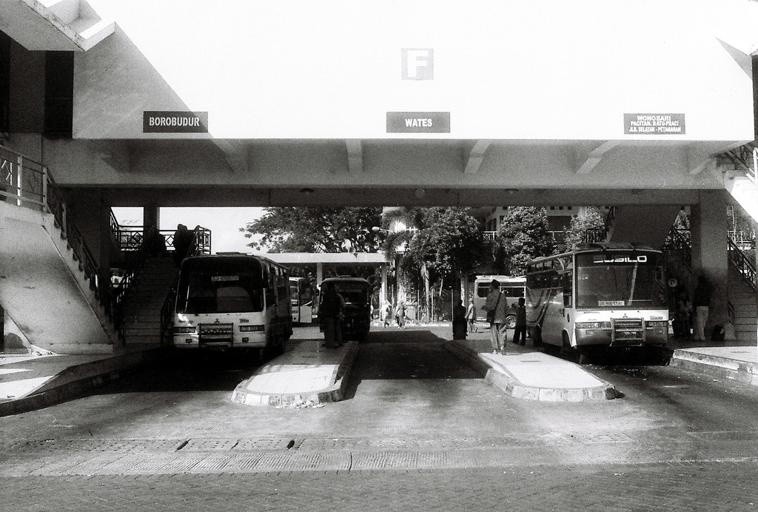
[465,298,478,332]
[513,297,526,346]
[380,300,390,327]
[694,274,711,341]
[396,300,407,329]
[460,299,468,336]
[394,302,402,327]
[480,278,507,355]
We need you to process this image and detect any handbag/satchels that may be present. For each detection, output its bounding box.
[487,310,495,323]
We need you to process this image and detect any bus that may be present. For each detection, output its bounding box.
[321,275,372,336]
[288,275,315,325]
[524,242,669,353]
[473,275,526,329]
[172,253,290,361]
